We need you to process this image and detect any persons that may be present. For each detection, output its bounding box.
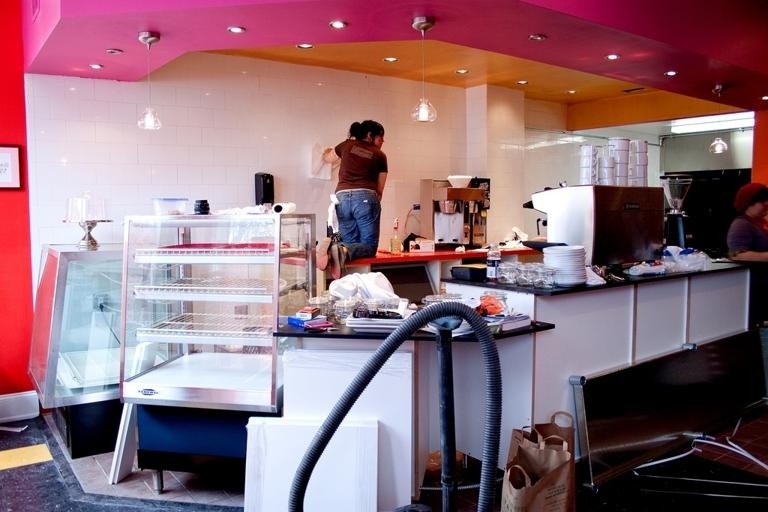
[727,182,768,262]
[314,120,388,280]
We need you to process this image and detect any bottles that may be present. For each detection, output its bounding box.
[390,218,401,253]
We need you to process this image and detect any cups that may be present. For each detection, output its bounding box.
[578,145,598,185]
[486,290,508,313]
[423,295,443,308]
[598,156,615,185]
[321,291,332,315]
[496,260,554,286]
[443,294,462,303]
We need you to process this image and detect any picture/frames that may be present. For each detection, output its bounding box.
[0,144,24,192]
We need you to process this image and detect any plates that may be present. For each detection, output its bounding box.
[346,309,416,334]
[543,247,588,288]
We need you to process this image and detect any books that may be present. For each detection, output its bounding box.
[480,313,531,331]
[419,321,502,337]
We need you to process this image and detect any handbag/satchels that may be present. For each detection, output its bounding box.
[498,435,576,511]
[508,411,576,495]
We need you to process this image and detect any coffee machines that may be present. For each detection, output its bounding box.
[419,179,490,252]
[523,185,665,273]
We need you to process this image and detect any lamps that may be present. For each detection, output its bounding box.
[411,14,439,124]
[137,29,165,131]
[709,89,729,157]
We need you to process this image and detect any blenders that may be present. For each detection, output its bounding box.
[658,175,693,248]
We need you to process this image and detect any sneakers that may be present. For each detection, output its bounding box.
[330,245,345,280]
[317,236,332,271]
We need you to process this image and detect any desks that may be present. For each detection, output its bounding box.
[290,239,545,278]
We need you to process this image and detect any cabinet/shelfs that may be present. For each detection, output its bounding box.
[26,242,140,403]
[116,212,297,411]
[439,259,749,470]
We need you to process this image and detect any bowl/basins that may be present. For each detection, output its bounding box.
[607,135,630,187]
[151,197,189,215]
[446,175,472,187]
[629,139,649,187]
